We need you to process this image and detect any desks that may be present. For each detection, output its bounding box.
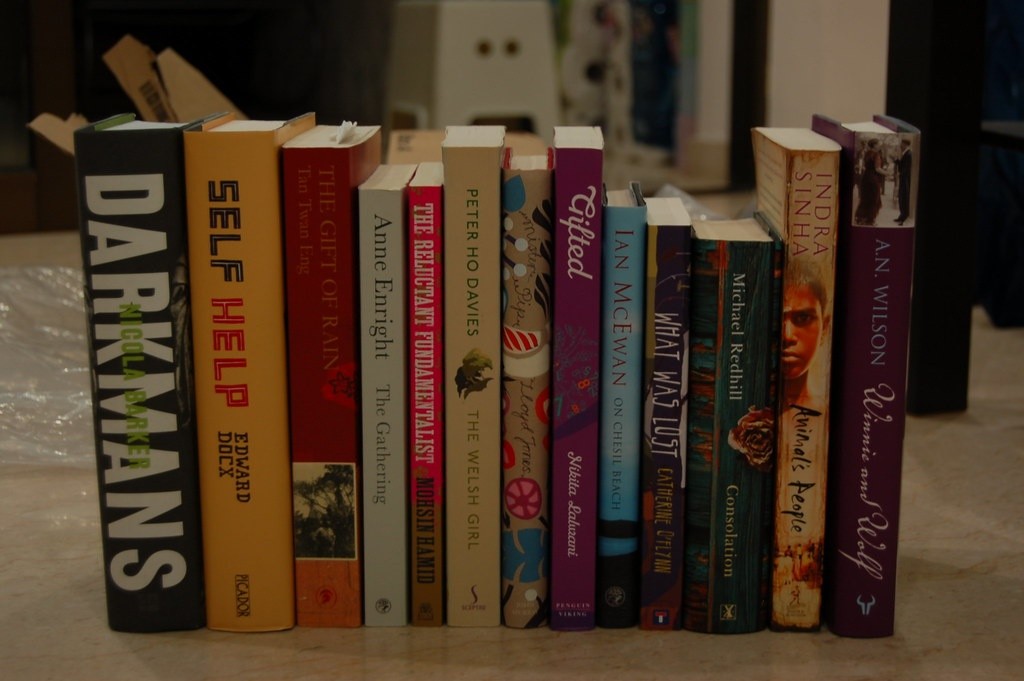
[0,228,1024,681]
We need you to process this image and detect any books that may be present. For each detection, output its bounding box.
[72,115,921,641]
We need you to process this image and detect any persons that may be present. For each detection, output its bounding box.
[777,262,828,546]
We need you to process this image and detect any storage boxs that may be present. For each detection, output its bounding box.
[26,37,251,158]
[387,129,545,164]
